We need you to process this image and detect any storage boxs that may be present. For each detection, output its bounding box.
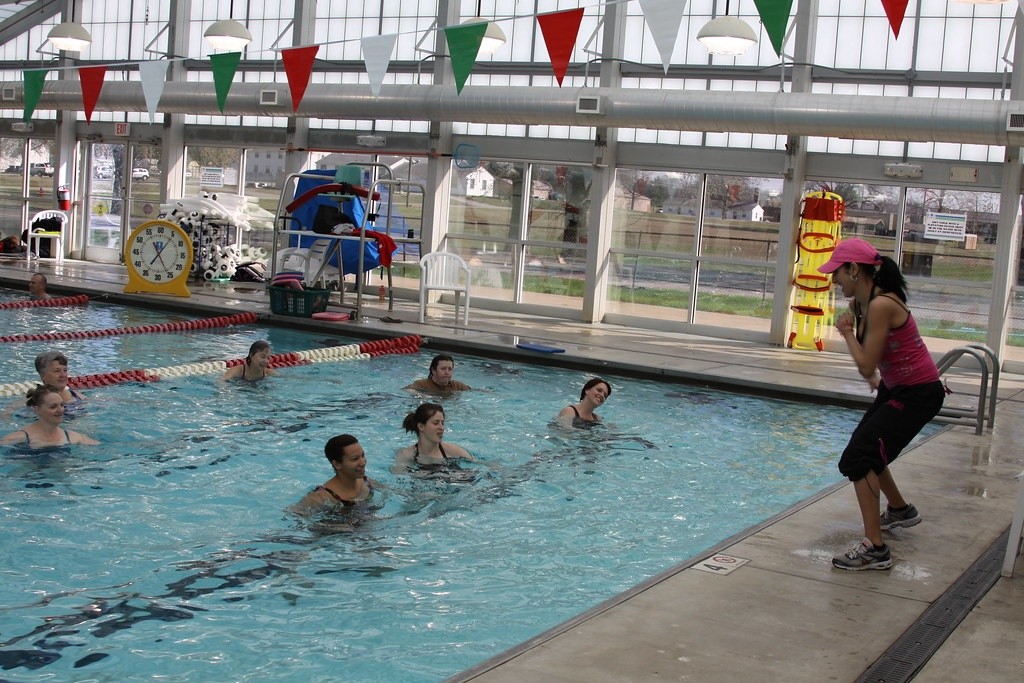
[266,284,333,317]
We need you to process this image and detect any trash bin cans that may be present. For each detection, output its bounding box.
[902,254,933,277]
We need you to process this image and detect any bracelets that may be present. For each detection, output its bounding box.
[840,329,853,335]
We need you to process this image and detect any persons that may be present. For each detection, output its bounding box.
[34,350,86,403]
[28,272,51,298]
[5,384,82,446]
[309,433,373,505]
[559,379,611,422]
[223,340,277,383]
[400,403,473,460]
[404,354,471,393]
[817,238,946,571]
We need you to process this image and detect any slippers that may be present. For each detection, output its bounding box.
[350,310,357,320]
[380,316,402,323]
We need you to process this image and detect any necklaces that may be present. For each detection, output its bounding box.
[859,312,863,318]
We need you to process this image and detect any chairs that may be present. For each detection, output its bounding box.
[286,239,332,289]
[27,209,69,264]
[419,252,471,326]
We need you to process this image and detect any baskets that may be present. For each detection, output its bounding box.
[268,285,331,317]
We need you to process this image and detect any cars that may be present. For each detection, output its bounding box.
[655,208,664,214]
[20,158,117,179]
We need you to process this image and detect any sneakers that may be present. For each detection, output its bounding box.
[879,502,923,529]
[832,536,893,570]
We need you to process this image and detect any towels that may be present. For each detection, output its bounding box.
[351,226,398,270]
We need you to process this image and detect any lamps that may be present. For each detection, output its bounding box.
[696,0,759,58]
[462,0,506,53]
[47,0,92,51]
[203,0,253,51]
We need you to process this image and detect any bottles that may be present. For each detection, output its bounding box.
[379,285,386,304]
[299,276,306,290]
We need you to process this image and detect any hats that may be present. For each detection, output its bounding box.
[816,238,881,274]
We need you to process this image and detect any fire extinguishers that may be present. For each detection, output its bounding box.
[57,183,70,210]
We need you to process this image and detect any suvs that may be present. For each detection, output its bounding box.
[132,168,150,182]
